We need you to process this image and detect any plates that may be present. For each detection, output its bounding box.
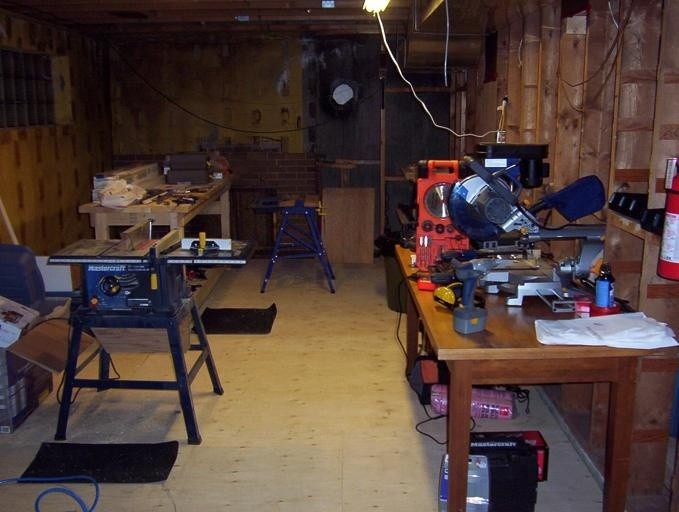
[190,241,219,252]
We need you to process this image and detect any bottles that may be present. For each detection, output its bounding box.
[595,262,614,307]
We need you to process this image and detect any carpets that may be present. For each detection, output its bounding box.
[18,440,178,483]
[193,302,278,334]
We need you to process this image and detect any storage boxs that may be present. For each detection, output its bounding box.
[0,295,40,347]
[0,319,95,435]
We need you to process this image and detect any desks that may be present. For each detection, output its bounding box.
[396,243,658,512]
[79,173,235,240]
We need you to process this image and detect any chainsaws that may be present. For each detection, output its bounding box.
[448,155,605,249]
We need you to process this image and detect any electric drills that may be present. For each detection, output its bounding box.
[453,261,486,334]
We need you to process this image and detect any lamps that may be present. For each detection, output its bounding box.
[362,0,390,14]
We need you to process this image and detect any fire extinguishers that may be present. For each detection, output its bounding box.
[657,158,679,282]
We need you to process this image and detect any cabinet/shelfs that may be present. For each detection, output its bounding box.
[54,297,224,444]
